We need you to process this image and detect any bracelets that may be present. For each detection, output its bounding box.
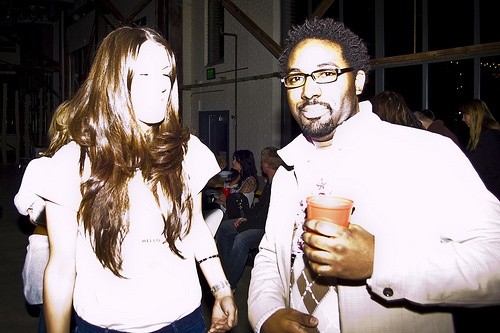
[211,279,230,293]
[198,254,219,265]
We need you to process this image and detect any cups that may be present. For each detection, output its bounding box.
[224,182,233,196]
[306,195,354,265]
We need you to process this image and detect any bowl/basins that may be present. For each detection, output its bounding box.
[219,171,232,177]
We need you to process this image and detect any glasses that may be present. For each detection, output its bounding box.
[281,67,355,89]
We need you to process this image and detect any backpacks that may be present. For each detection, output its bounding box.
[225,192,250,220]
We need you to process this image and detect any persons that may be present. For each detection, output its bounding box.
[33,25,238,333]
[247,18,500,333]
[13,102,75,333]
[202,90,500,307]
